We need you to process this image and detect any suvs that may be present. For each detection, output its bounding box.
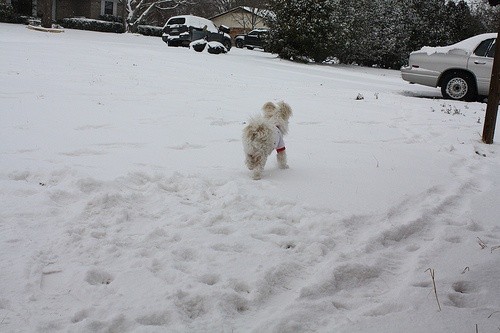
[235,29,278,52]
[162,15,232,51]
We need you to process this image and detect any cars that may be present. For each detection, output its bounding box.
[400,32,500,101]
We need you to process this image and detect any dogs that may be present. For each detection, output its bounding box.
[244,103,291,180]
